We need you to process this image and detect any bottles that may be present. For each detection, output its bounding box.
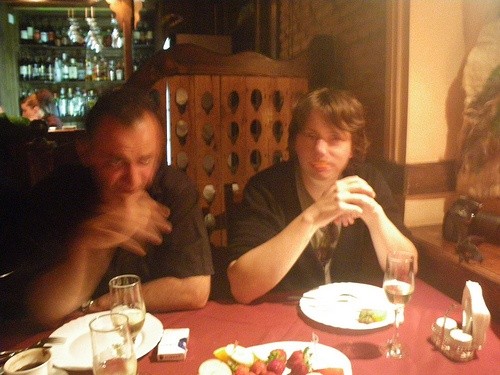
[19,15,124,121]
[133,15,154,72]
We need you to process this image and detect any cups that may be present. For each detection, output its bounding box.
[89,313,137,375]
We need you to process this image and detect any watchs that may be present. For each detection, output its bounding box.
[81,299,94,313]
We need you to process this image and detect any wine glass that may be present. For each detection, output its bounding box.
[380,250,416,361]
[109,275,147,345]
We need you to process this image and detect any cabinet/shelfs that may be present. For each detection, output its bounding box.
[20,10,155,122]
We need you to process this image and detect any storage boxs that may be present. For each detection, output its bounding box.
[157,328,190,360]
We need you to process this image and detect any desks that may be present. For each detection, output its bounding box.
[0,275,500,375]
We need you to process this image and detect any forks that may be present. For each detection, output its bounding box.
[0,336,66,358]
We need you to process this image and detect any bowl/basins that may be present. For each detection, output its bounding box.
[3,347,53,375]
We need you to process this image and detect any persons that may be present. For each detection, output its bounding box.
[18,85,215,330]
[20,89,59,125]
[226,85,418,302]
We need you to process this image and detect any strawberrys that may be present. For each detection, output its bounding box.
[230,349,315,375]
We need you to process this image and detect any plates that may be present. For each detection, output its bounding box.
[298,281,395,331]
[198,340,352,375]
[43,309,163,371]
[53,367,70,375]
